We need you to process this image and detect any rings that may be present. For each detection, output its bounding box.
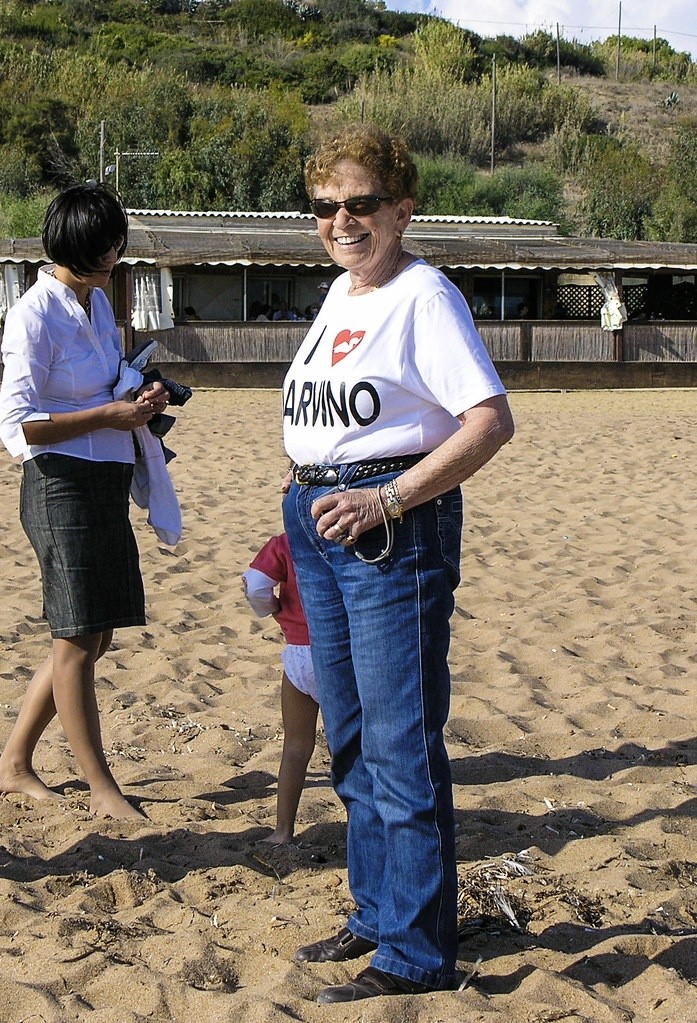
[333,523,344,535]
[346,535,357,544]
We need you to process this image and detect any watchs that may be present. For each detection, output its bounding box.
[385,482,402,519]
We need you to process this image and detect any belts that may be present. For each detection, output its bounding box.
[294,451,432,485]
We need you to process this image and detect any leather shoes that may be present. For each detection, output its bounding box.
[316,966,439,1004]
[295,927,378,962]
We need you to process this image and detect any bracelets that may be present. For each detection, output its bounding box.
[391,479,402,510]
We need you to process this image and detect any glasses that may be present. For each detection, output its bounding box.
[308,195,391,219]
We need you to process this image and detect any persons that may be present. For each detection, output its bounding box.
[1,183,172,820]
[277,120,518,1003]
[180,279,330,321]
[242,527,324,845]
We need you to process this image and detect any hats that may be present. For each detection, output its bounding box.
[316,282,329,289]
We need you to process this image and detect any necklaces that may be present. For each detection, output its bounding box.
[349,247,406,295]
[49,267,91,318]
